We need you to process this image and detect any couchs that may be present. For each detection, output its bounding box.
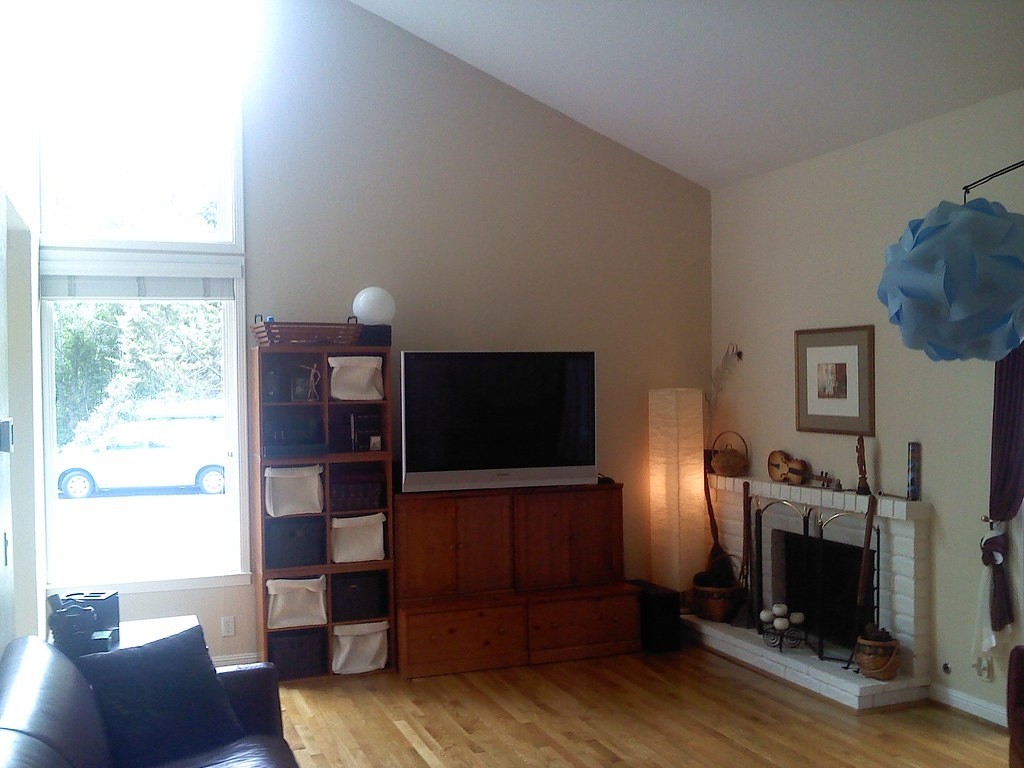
[0,635,298,768]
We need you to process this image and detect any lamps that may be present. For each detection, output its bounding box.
[354,285,396,346]
[650,388,706,591]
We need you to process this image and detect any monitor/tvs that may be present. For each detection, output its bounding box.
[401,349,598,491]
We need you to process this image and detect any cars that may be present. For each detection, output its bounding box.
[56,419,226,497]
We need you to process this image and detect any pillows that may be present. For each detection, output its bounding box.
[79,626,248,768]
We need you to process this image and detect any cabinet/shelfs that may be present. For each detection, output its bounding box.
[392,482,645,677]
[249,345,397,685]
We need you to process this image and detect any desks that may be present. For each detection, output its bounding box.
[47,612,200,651]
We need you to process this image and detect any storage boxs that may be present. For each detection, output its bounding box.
[51,590,122,642]
[333,620,390,674]
[332,573,388,621]
[266,574,328,629]
[266,521,326,568]
[269,630,329,679]
[331,512,386,563]
[331,467,384,510]
[263,464,324,517]
[327,356,384,401]
[263,413,327,457]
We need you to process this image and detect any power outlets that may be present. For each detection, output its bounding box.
[222,615,235,637]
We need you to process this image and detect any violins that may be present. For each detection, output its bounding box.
[767,450,834,488]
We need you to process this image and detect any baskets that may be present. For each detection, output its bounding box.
[330,479,381,511]
[853,635,901,681]
[270,632,323,679]
[692,585,733,623]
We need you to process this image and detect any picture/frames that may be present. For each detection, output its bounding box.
[794,324,876,438]
